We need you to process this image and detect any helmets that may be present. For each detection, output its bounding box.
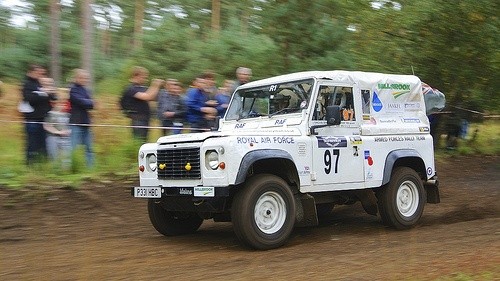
[273,89,298,113]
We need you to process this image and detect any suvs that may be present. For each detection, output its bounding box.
[130,64,456,251]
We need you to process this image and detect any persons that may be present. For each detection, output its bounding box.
[159,67,254,136]
[119,65,163,145]
[22,65,98,174]
[273,89,299,113]
